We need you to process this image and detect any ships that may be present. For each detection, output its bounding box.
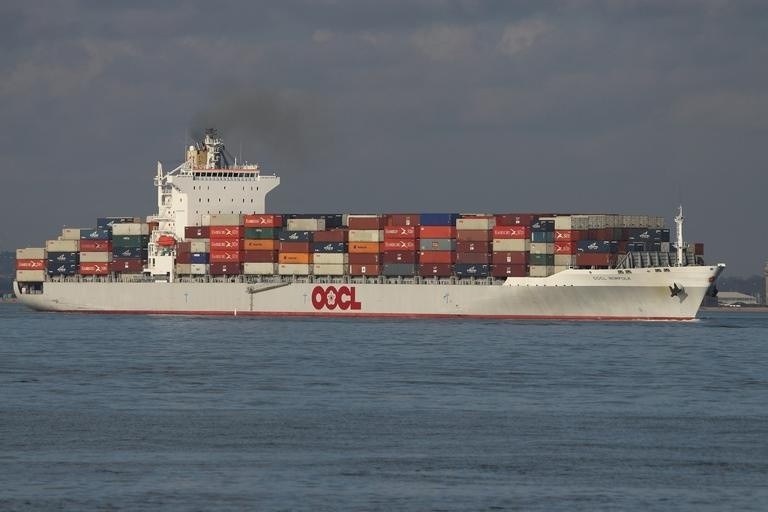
[13,127,726,321]
